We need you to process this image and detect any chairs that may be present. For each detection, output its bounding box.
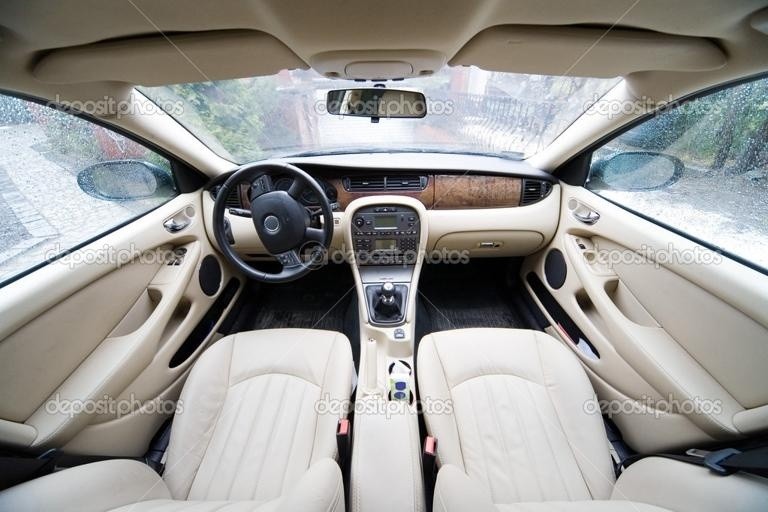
[0,327,357,511]
[417,327,768,512]
